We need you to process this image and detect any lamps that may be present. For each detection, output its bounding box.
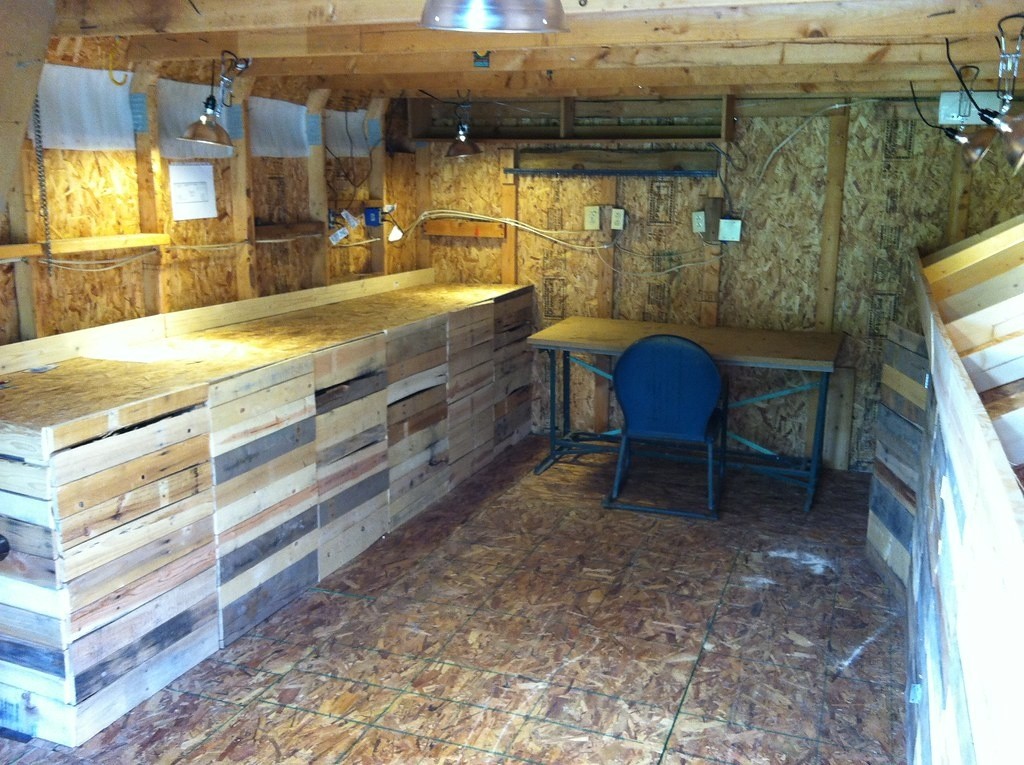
[910,81,1001,169]
[945,37,1024,177]
[444,103,483,158]
[176,58,233,148]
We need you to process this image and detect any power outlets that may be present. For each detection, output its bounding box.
[692,211,705,233]
[584,206,600,230]
[611,209,624,229]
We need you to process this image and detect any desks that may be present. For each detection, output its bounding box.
[526,315,846,508]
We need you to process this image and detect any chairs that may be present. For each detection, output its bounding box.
[609,334,721,521]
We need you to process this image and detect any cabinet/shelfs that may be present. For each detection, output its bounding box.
[0,284,534,748]
